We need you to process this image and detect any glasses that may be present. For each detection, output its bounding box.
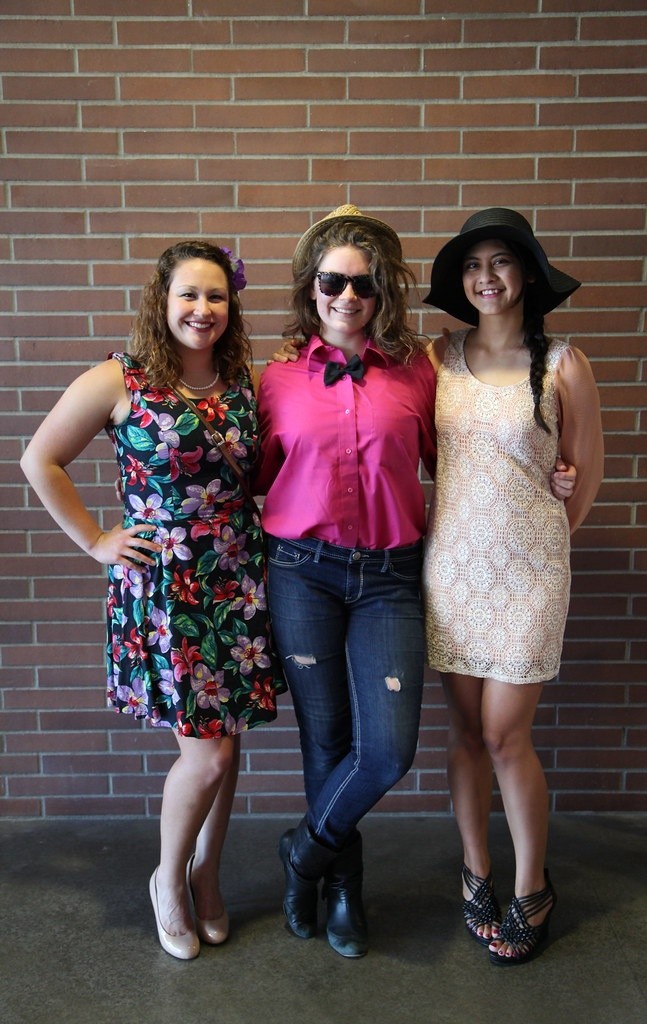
[315,270,386,300]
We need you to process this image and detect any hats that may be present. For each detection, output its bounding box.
[421,207,581,329]
[291,204,403,285]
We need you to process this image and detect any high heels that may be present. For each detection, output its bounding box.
[149,864,201,960]
[186,852,231,944]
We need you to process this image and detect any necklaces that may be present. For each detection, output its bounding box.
[178,365,219,390]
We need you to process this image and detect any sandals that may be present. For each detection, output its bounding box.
[487,882,558,967]
[460,862,503,947]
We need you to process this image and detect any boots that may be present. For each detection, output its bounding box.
[277,813,341,939]
[322,830,370,957]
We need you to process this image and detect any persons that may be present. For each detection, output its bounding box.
[244,203,583,958]
[266,209,604,967]
[21,240,289,959]
